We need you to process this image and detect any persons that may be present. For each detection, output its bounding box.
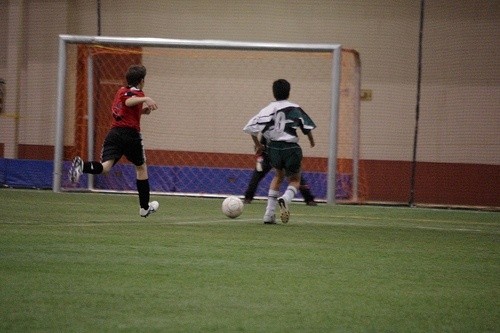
[68,65,160,219]
[243,79,317,225]
[241,136,318,206]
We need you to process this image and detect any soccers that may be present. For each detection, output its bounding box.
[221,196,244,218]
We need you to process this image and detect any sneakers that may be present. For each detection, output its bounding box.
[140,201,159,218]
[67,156,84,188]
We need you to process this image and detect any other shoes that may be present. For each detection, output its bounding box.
[264,215,275,223]
[306,199,317,206]
[243,198,251,204]
[277,198,290,224]
